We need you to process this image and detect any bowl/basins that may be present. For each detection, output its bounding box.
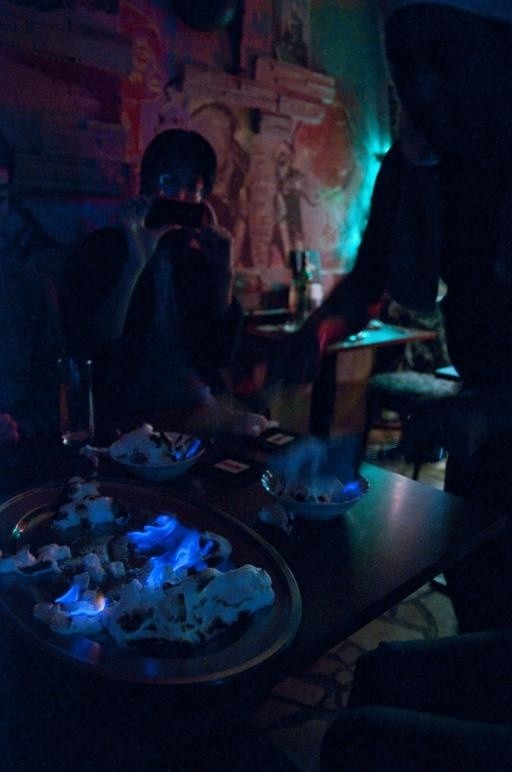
[261,463,369,522]
[108,431,206,482]
[0,481,302,685]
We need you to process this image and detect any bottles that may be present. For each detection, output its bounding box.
[284,270,309,333]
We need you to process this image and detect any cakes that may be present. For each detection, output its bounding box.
[3,477,275,658]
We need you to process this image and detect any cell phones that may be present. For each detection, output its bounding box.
[149,199,205,227]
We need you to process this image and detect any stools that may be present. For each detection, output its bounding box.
[359,369,460,480]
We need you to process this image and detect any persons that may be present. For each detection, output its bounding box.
[280,1,509,632]
[62,127,272,438]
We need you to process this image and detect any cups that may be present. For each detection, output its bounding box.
[57,357,95,448]
